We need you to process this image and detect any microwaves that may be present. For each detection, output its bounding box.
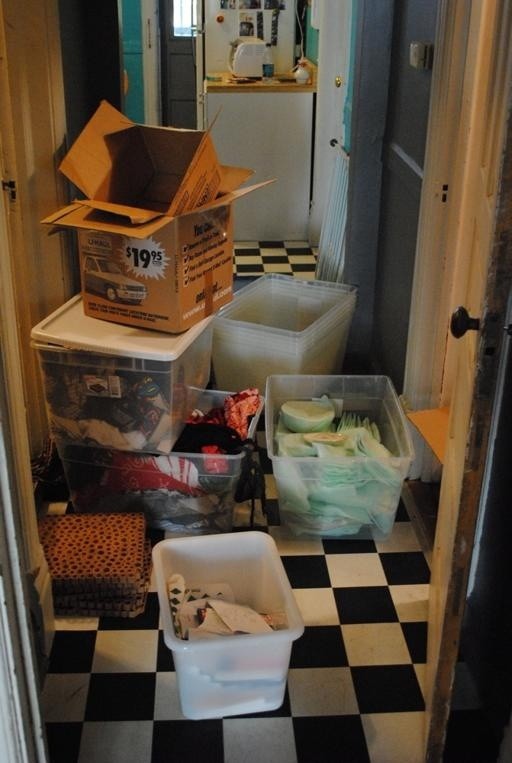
[227,36,267,78]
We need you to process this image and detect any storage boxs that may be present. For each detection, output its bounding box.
[54,388,266,534]
[264,373,416,541]
[212,271,360,415]
[28,290,212,454]
[151,530,306,721]
[39,98,279,335]
[70,103,224,226]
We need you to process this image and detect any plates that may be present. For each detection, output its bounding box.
[303,431,349,446]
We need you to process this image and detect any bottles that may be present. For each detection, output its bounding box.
[260,41,276,85]
[294,58,310,85]
